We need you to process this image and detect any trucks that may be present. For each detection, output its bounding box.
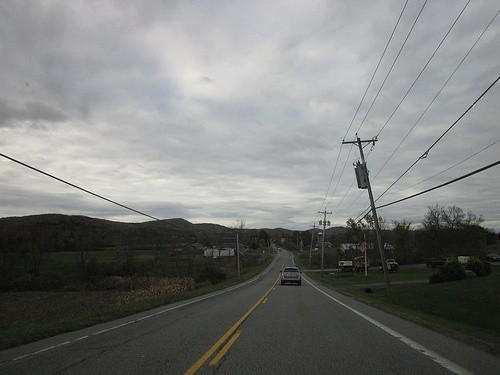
[337,256,370,272]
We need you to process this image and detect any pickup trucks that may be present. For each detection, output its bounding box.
[280,267,302,286]
[379,259,399,273]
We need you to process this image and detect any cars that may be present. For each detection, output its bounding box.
[426,257,452,268]
[480,252,500,261]
